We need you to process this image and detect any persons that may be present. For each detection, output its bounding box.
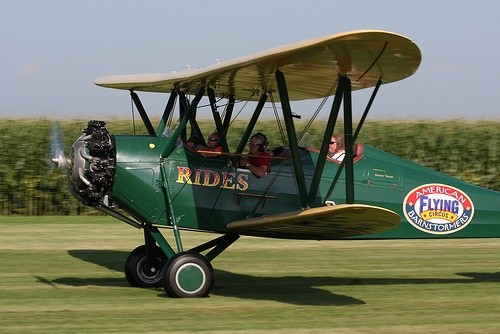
[186,132,223,158]
[235,133,270,178]
[327,134,346,162]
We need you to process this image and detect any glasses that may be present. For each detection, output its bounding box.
[208,137,218,141]
[330,142,336,144]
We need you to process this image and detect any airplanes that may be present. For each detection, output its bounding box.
[51,29,500,298]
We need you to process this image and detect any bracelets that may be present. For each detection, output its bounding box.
[244,162,251,167]
[192,143,198,150]
